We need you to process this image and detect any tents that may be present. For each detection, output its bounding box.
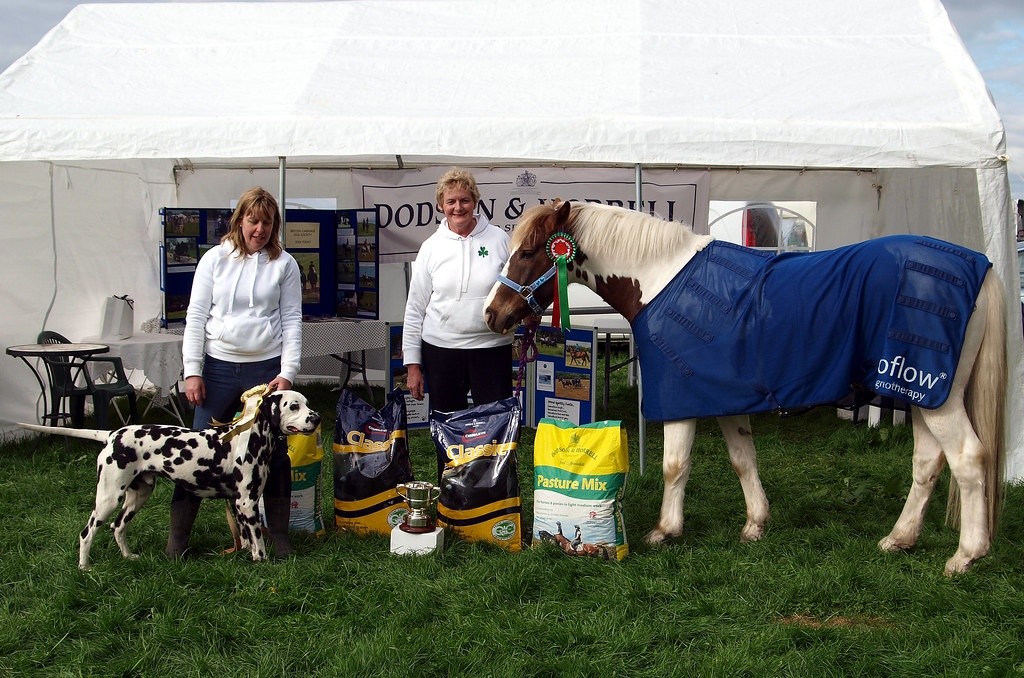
[0,0,1024,485]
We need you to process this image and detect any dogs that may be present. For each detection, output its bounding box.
[2,386,323,575]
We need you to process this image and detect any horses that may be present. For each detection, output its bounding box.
[538,530,611,560]
[480,200,1010,584]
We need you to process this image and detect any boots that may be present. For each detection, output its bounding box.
[268,514,293,559]
[165,516,195,560]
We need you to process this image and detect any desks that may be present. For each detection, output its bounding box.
[593,319,634,412]
[69,333,185,427]
[136,318,386,417]
[6,345,111,448]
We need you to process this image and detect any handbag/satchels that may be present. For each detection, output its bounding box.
[98,294,134,340]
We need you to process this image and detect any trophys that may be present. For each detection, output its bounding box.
[396,481,442,534]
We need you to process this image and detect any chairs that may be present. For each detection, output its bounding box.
[38,330,140,444]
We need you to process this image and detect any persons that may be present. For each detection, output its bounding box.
[164,186,302,564]
[402,167,512,487]
